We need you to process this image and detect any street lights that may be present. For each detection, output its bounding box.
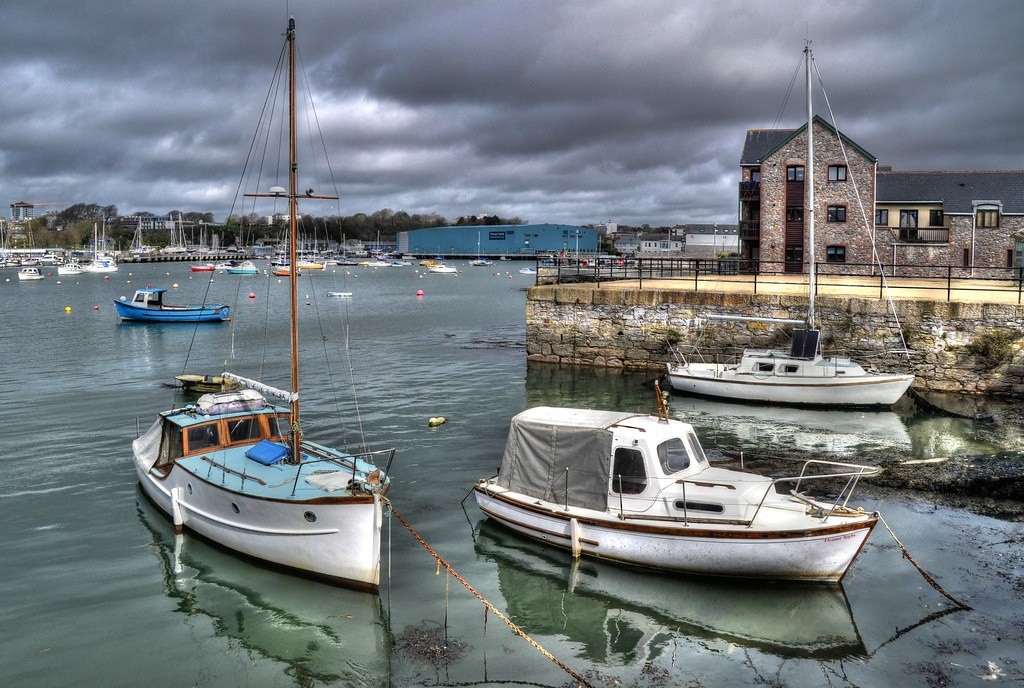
[576,230,579,266]
[713,222,717,256]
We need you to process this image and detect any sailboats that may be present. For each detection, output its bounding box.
[468,230,494,266]
[131,15,396,592]
[435,246,445,260]
[666,21,916,408]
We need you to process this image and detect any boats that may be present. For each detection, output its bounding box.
[427,261,457,273]
[114,284,230,323]
[500,257,512,261]
[58,263,84,275]
[518,267,537,275]
[17,267,45,279]
[460,406,879,583]
[0,213,406,276]
[585,254,636,268]
[419,260,438,266]
[134,481,394,688]
[473,518,870,668]
[402,256,416,260]
